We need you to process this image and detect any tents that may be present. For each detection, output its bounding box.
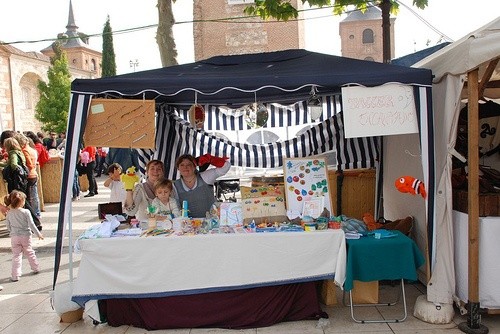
[51,48,435,288]
[408,16,500,306]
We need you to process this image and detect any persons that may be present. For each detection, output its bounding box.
[4,189,44,282]
[72,146,111,200]
[150,177,181,218]
[123,159,166,220]
[0,129,66,231]
[169,154,230,219]
[104,163,126,202]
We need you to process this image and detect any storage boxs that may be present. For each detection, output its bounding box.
[454,188,500,216]
[321,280,379,305]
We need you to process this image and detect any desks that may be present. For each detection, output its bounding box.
[71,223,347,330]
[341,229,426,324]
[452,209,499,317]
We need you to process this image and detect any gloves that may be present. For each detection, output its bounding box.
[198,153,225,167]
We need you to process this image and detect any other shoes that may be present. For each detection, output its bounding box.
[10,275,20,281]
[72,196,80,200]
[84,191,95,197]
